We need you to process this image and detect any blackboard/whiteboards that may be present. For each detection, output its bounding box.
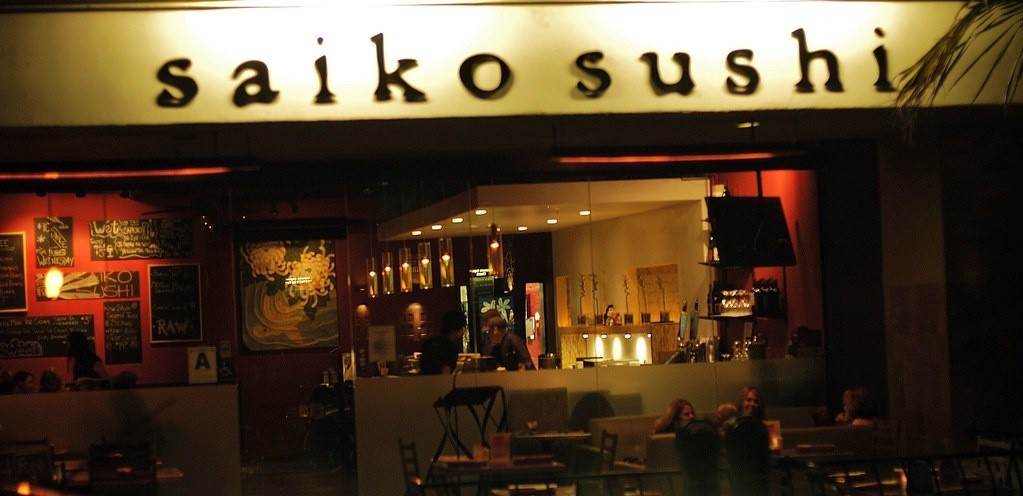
[88,218,195,260]
[35,270,140,301]
[148,264,203,343]
[34,216,74,267]
[0,231,29,312]
[103,300,143,365]
[0,313,96,359]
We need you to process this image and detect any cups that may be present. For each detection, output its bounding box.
[576,361,583,369]
[492,433,510,469]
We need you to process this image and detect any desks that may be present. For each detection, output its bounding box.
[778,448,855,496]
[50,458,185,496]
[516,433,592,483]
[433,459,647,496]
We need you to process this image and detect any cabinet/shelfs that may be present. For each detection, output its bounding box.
[698,217,789,361]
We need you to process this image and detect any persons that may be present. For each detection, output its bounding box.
[419,310,465,376]
[652,384,764,436]
[479,309,537,370]
[0,331,138,396]
[810,382,870,427]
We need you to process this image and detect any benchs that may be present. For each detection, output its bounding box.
[591,407,874,496]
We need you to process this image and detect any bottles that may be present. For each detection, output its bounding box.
[711,276,781,318]
[323,367,339,386]
[673,300,767,363]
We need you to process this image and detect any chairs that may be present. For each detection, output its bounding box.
[868,420,1023,496]
[0,435,158,496]
[398,429,619,496]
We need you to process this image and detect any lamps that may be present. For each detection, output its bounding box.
[381,181,395,294]
[417,179,433,290]
[365,187,378,298]
[485,173,504,278]
[439,177,456,287]
[398,188,413,293]
